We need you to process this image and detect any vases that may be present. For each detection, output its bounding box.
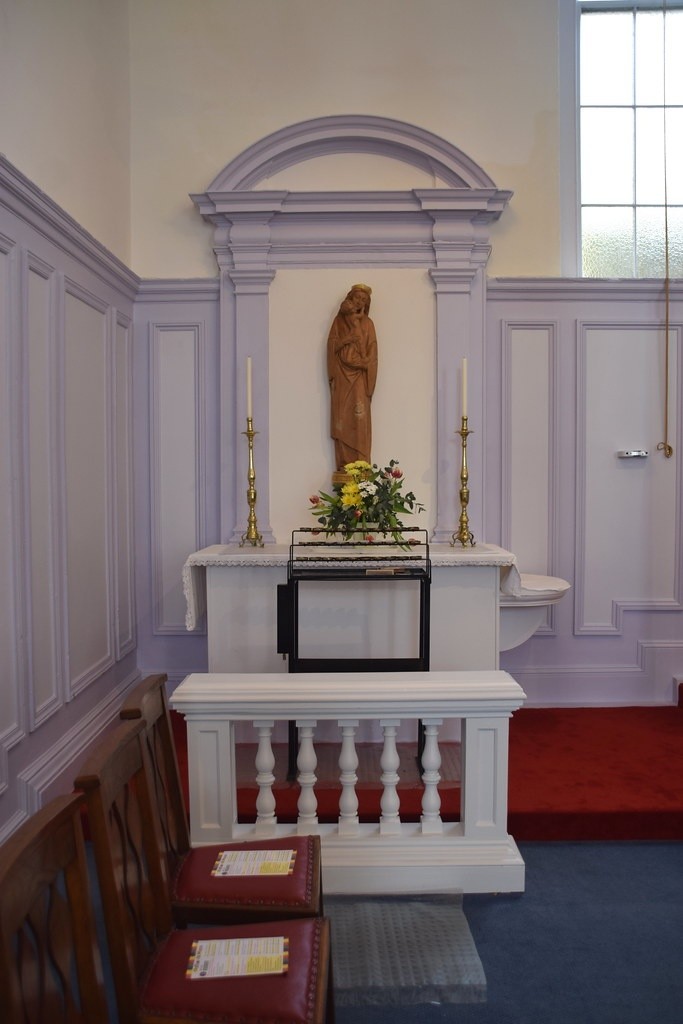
[353,521,378,543]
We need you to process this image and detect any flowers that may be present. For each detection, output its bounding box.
[309,456,426,551]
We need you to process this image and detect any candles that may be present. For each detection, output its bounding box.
[462,357,467,418]
[247,356,252,418]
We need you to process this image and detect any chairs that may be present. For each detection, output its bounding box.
[0,672,334,1024]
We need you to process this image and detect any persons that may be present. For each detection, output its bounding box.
[327,282,378,471]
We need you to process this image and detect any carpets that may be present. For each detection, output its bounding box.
[323,891,489,1005]
